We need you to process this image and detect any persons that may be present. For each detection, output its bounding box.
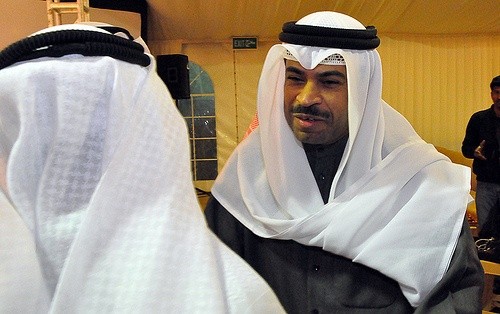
[462,74,500,313]
[200,9,488,314]
[0,22,287,314]
